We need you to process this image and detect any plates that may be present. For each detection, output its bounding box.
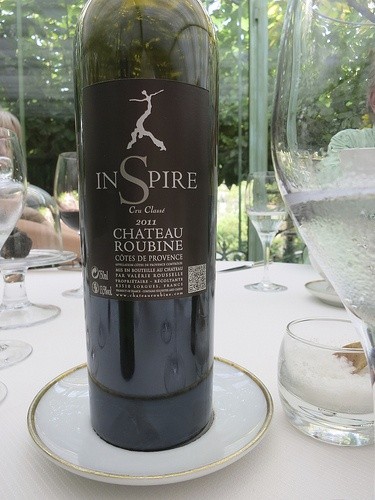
[27,354,274,486]
[304,277,349,308]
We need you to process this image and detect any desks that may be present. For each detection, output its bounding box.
[0,261,375,500]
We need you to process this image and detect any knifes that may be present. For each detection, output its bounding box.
[218,260,273,273]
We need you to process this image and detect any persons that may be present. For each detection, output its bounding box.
[0,110,85,271]
[328,77,375,175]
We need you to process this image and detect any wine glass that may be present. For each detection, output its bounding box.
[0,128,34,368]
[53,151,92,298]
[242,172,287,292]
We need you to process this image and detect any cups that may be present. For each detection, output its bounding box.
[274,319,375,448]
[268,0,375,392]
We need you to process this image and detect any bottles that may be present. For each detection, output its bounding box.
[74,1,222,449]
